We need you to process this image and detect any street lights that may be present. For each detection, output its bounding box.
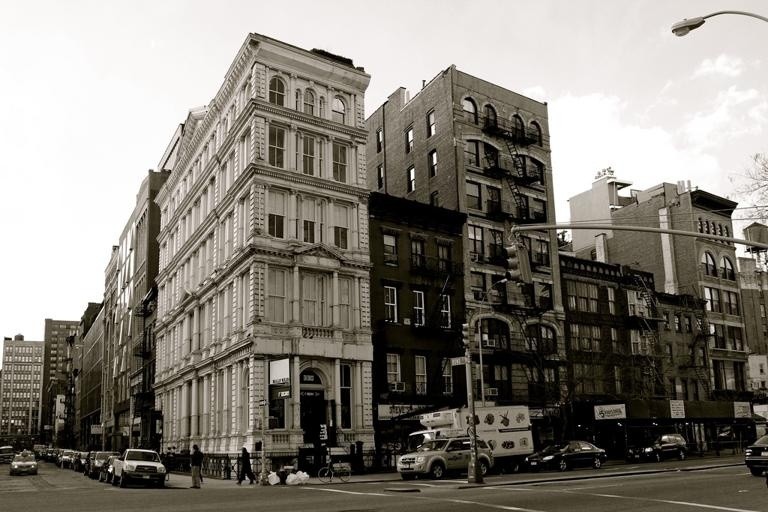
[477,278,508,407]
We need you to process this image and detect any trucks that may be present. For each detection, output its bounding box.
[406,405,536,465]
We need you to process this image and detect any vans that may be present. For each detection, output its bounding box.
[0,445,14,464]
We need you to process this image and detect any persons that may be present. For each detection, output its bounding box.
[235,447,255,486]
[189,444,204,489]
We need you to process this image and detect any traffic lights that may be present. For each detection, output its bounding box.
[502,242,532,285]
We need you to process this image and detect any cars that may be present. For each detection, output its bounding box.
[10,451,38,475]
[524,439,608,471]
[744,435,767,475]
[396,438,495,481]
[624,433,687,464]
[46,447,167,487]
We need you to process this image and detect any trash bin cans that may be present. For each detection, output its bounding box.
[299,448,326,477]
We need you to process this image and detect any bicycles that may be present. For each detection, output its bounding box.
[317,457,351,481]
